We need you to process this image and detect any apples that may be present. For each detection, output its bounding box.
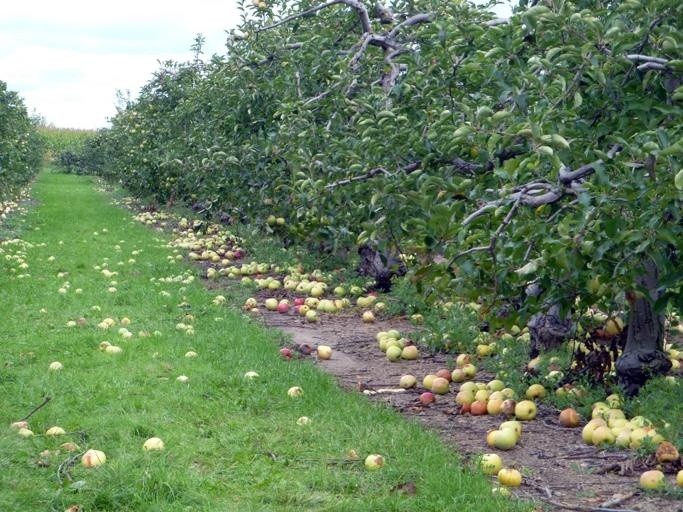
[0,0,683,473]
